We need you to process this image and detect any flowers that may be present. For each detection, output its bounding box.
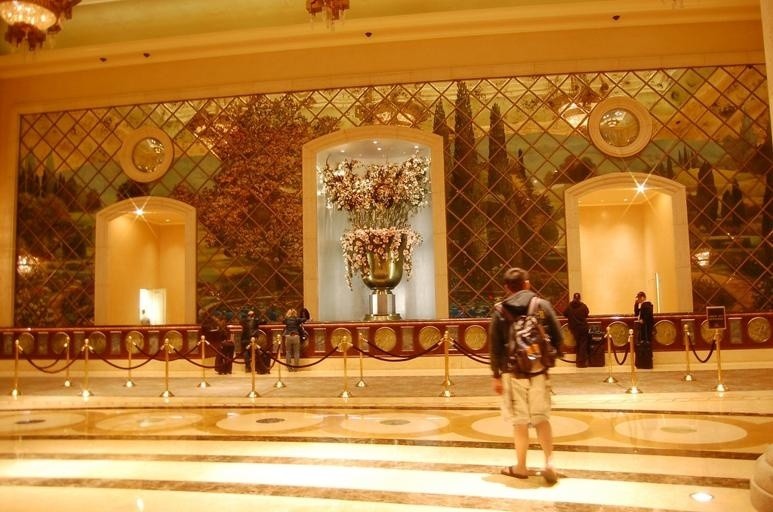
[316,142,433,295]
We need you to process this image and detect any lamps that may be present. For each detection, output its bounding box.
[14,253,40,283]
[0,0,81,59]
[303,0,352,34]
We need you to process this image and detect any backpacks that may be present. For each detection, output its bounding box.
[493,296,558,375]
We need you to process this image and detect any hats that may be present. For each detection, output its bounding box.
[636,292,645,298]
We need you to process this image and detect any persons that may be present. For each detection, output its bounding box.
[277,308,308,371]
[634,291,654,341]
[564,290,590,368]
[195,309,227,374]
[237,311,265,371]
[487,266,566,486]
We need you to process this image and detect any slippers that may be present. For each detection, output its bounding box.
[501,466,529,478]
[541,469,557,483]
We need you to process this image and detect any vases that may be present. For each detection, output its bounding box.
[351,237,407,323]
